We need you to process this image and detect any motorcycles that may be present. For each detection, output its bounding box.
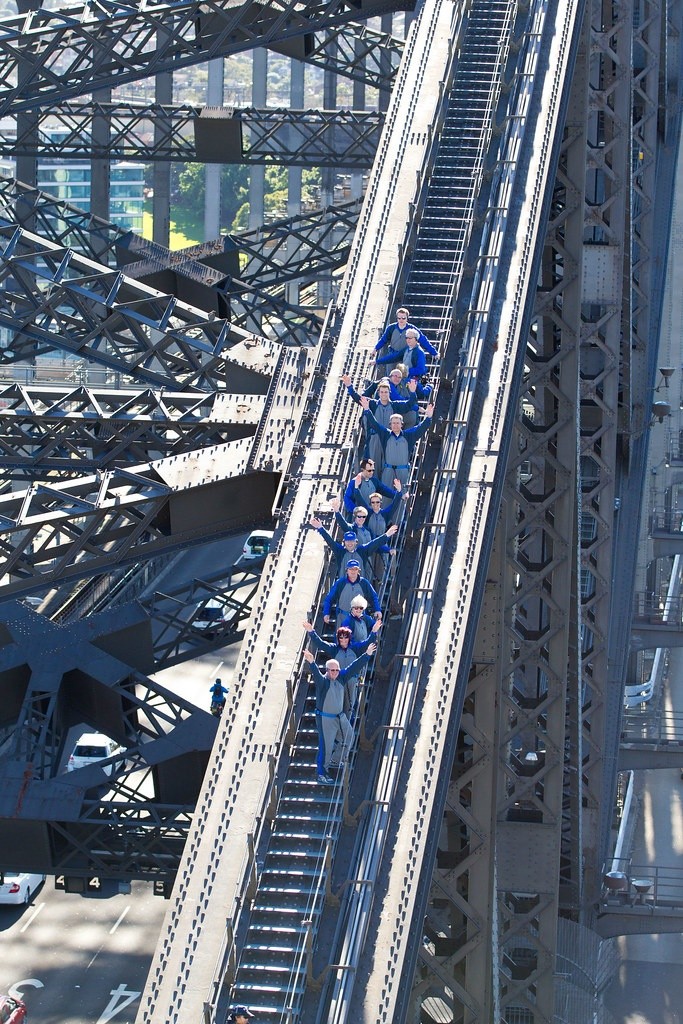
[210,688,229,718]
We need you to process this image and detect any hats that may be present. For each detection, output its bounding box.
[346,559,360,569]
[343,531,357,540]
[232,1005,255,1018]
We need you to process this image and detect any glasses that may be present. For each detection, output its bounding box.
[405,337,414,339]
[352,607,364,610]
[327,668,340,672]
[339,636,349,639]
[371,501,381,504]
[397,316,407,320]
[363,469,375,472]
[355,515,366,519]
[241,1016,247,1019]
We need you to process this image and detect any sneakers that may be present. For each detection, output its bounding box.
[330,759,344,766]
[318,774,333,784]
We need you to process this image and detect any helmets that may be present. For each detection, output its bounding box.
[216,679,221,683]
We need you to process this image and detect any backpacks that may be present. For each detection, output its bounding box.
[215,686,221,695]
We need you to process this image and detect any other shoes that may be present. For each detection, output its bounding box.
[333,739,346,749]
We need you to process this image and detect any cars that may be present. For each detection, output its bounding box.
[67,731,127,776]
[0,995,28,1024]
[189,596,240,634]
[243,530,274,560]
[0,872,46,907]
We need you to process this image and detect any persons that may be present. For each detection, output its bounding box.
[332,499,397,582]
[394,363,434,430]
[369,308,441,377]
[355,474,403,567]
[373,369,426,416]
[303,642,377,785]
[302,619,383,723]
[323,559,383,644]
[210,679,229,712]
[226,1005,255,1024]
[341,594,382,646]
[310,518,398,579]
[344,459,409,513]
[343,373,418,479]
[360,396,434,527]
[351,328,426,403]
